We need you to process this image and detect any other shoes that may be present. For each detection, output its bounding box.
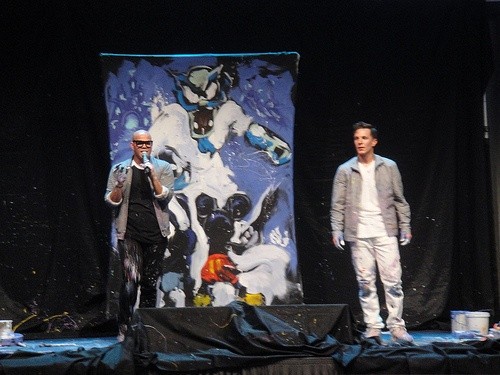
[118,321,128,335]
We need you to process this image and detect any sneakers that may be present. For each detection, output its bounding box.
[390,326,414,343]
[364,327,381,343]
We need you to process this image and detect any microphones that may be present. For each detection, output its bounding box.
[141,152,150,174]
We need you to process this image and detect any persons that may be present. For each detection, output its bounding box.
[104,130,174,342]
[331,122,414,344]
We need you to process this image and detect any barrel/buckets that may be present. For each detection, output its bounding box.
[466,312,490,335]
[451,311,468,337]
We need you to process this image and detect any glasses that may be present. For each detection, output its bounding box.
[133,141,153,148]
